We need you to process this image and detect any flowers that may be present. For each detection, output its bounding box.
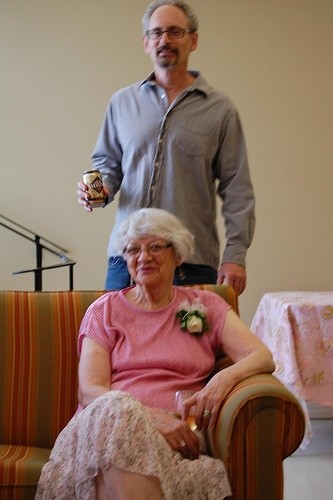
[176,295,210,341]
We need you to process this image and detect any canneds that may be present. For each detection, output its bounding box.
[83,169,106,208]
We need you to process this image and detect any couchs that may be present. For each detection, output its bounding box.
[1,285,306,500]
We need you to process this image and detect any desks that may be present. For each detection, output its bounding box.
[249,290,333,450]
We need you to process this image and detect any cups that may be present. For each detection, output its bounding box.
[175,390,198,432]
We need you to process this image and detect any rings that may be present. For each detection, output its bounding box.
[203,409,211,418]
[178,439,186,447]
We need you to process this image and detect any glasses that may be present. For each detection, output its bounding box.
[121,243,173,255]
[147,27,194,41]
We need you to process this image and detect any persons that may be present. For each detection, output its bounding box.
[76,0,255,298]
[35,208,276,500]
